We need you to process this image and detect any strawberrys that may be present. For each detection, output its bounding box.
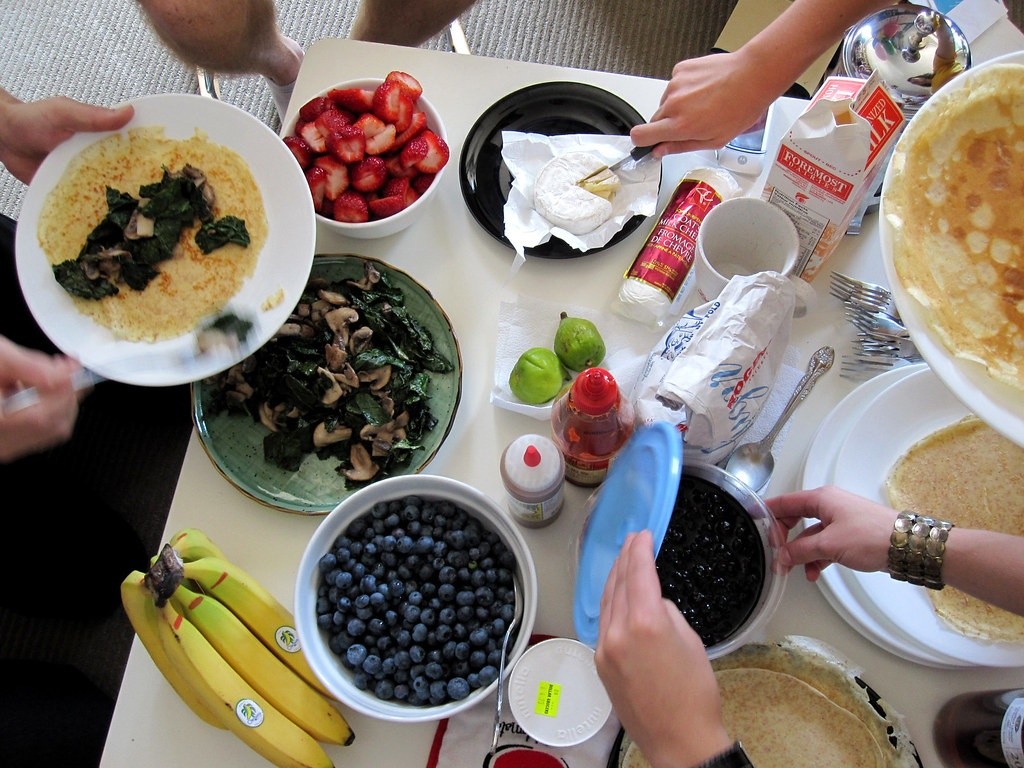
[281,71,451,224]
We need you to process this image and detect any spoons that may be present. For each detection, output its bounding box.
[723,346,835,494]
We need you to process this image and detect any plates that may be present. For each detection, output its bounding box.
[189,251,465,517]
[794,362,1024,671]
[14,92,317,387]
[879,46,1024,452]
[508,637,614,748]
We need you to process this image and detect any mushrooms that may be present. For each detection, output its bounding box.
[225,260,411,482]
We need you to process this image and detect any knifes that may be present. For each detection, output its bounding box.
[607,142,664,171]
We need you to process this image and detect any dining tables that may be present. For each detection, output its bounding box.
[97,40,1024,768]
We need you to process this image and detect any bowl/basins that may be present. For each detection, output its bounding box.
[280,78,448,240]
[293,473,538,724]
[564,461,790,663]
[458,80,662,259]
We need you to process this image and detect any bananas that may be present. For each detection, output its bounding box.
[120,528,355,768]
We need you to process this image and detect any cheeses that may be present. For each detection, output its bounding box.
[533,153,621,235]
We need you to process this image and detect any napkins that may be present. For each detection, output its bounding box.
[500,128,664,273]
[489,290,643,420]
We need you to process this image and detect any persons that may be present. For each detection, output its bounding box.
[593,486,1024,768]
[630,0,899,159]
[0,94,193,768]
[137,0,475,124]
[852,39,932,106]
[908,9,963,95]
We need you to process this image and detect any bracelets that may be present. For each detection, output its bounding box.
[887,509,955,590]
[692,741,755,768]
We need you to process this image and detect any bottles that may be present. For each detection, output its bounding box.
[499,432,566,529]
[548,367,637,490]
[932,687,1024,768]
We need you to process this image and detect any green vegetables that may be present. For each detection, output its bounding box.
[207,272,455,489]
[50,165,252,300]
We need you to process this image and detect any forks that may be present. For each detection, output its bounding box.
[829,270,923,383]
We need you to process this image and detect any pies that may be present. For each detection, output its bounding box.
[618,631,922,768]
[885,63,1024,394]
[882,415,1024,643]
[38,125,268,344]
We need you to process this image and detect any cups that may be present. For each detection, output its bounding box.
[828,5,972,135]
[694,196,801,301]
